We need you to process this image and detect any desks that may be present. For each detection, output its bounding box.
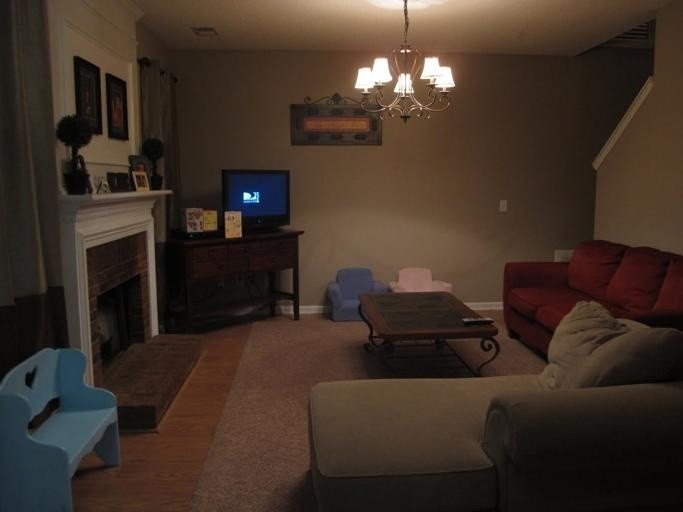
[162,214,305,333]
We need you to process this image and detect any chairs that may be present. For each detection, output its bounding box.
[0,348,121,512]
[389,268,451,292]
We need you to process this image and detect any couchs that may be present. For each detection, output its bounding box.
[503,240,683,356]
[307,342,682,511]
[326,268,390,323]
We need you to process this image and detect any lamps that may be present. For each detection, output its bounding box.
[348,0,485,135]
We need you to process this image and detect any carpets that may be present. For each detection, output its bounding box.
[191,322,547,511]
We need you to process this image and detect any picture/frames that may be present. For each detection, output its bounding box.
[132,171,150,192]
[74,55,102,135]
[106,73,128,139]
[290,93,381,145]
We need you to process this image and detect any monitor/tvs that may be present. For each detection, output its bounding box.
[221,168,291,234]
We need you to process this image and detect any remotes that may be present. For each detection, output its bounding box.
[462,317,495,325]
